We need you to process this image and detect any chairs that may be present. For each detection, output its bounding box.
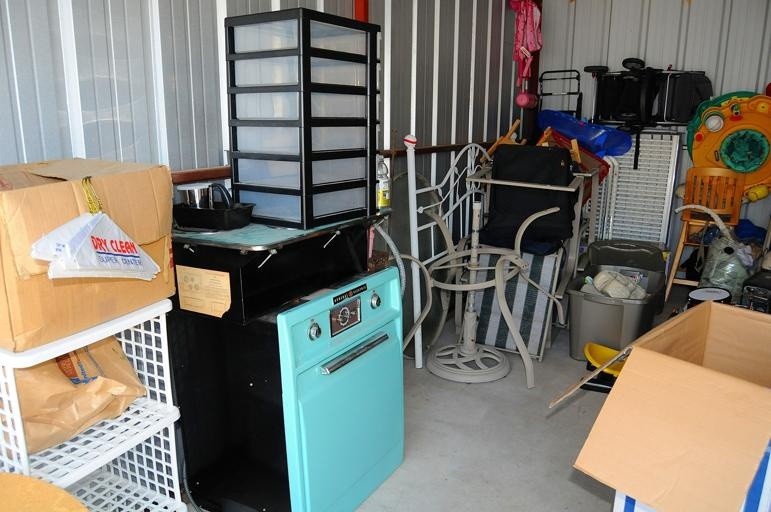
[663,167,746,304]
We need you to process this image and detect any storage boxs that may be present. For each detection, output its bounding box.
[564,262,666,362]
[549,301,771,512]
[0,157,178,354]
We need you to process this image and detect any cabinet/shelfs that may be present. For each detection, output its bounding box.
[222,7,382,231]
[0,298,190,512]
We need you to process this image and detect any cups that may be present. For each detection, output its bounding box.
[176,182,233,209]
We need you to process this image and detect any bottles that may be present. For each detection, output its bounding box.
[375,155,391,210]
